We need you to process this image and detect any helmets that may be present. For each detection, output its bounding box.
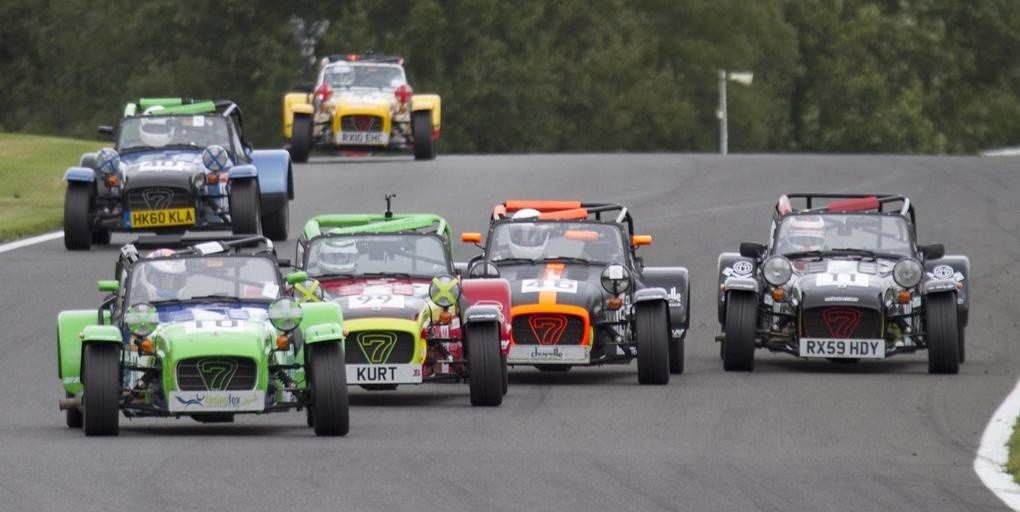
[330,60,356,87]
[315,236,358,276]
[784,209,826,254]
[140,249,187,301]
[508,209,548,258]
[139,104,175,148]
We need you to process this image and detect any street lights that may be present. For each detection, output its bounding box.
[717,65,756,156]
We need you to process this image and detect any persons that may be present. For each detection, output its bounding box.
[776,207,830,257]
[133,102,176,147]
[131,248,195,299]
[496,210,555,262]
[311,229,360,280]
[324,59,357,85]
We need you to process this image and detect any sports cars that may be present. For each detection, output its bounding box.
[281,45,445,162]
